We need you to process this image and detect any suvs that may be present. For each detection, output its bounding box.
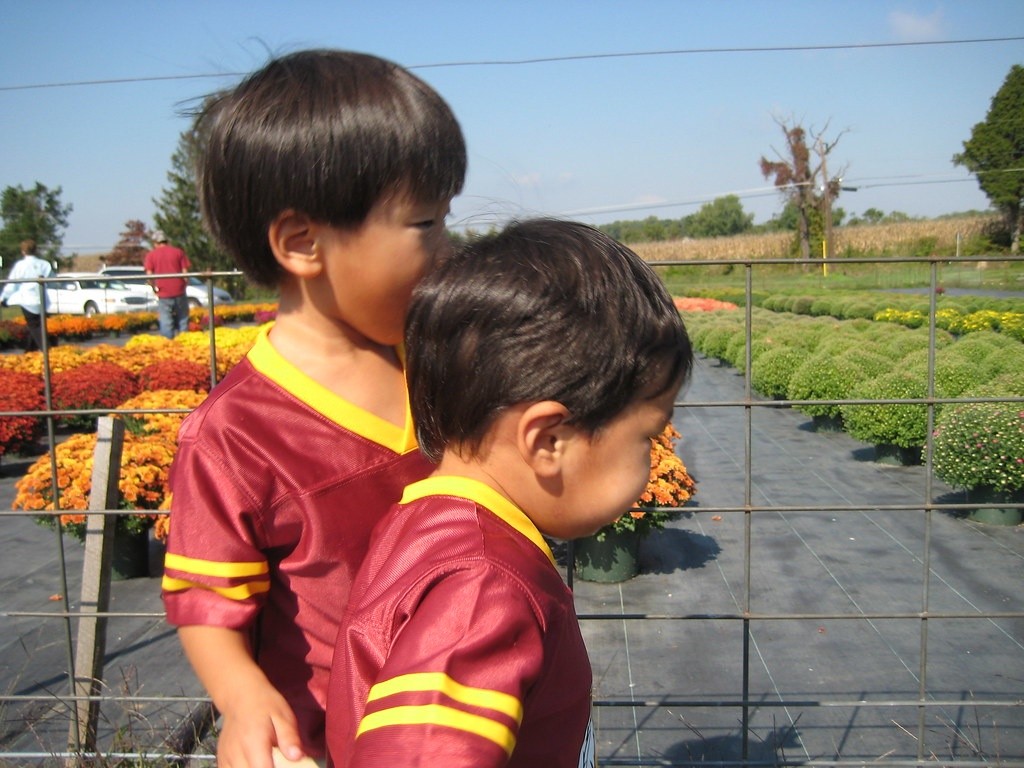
[96,265,232,314]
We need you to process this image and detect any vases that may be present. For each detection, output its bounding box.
[577,529,642,583]
[966,484,1024,525]
[873,444,916,466]
[813,416,842,435]
[109,528,150,581]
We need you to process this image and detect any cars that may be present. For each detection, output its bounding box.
[45,273,160,319]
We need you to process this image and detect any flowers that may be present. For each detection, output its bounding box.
[670,290,1024,499]
[0,302,697,542]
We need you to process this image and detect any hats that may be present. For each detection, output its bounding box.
[151,230,168,242]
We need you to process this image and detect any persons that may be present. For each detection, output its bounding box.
[0,239,56,353]
[322,216,696,768]
[144,231,191,339]
[158,50,469,768]
